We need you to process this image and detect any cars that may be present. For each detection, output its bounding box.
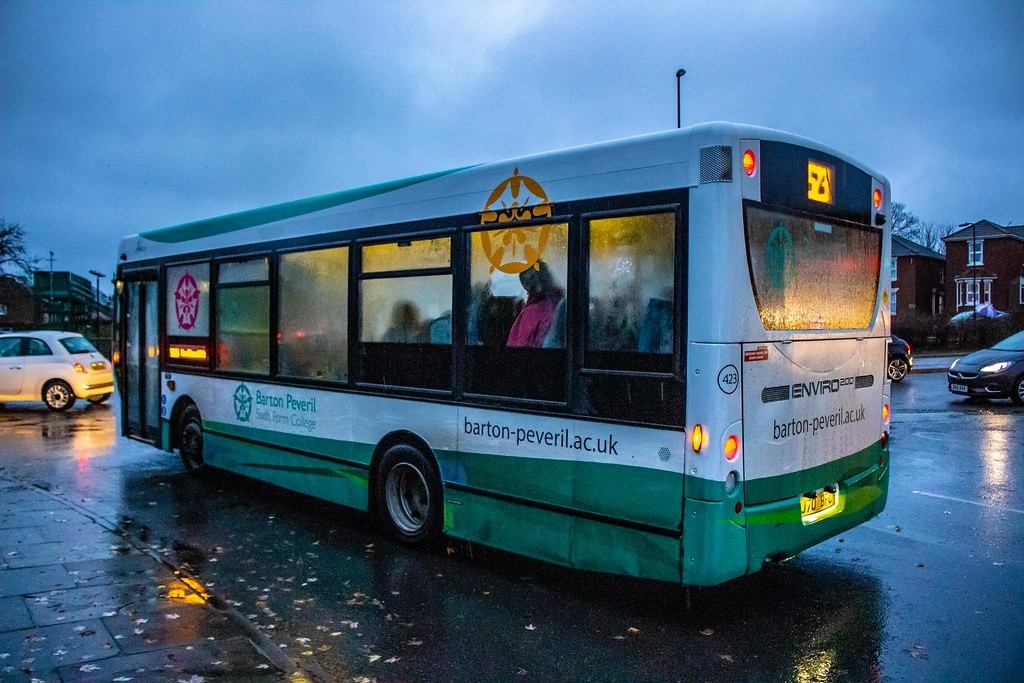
[947,329,1024,409]
[0,329,115,413]
[884,333,913,384]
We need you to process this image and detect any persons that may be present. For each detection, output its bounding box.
[507,260,565,349]
[380,300,425,344]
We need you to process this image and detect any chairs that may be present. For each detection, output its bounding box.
[541,296,605,351]
[428,315,452,344]
[637,298,674,354]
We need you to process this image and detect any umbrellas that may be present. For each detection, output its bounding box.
[975,303,997,319]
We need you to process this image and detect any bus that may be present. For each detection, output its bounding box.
[107,120,893,593]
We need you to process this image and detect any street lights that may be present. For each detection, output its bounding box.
[89,268,106,354]
[675,68,687,130]
[959,221,978,353]
